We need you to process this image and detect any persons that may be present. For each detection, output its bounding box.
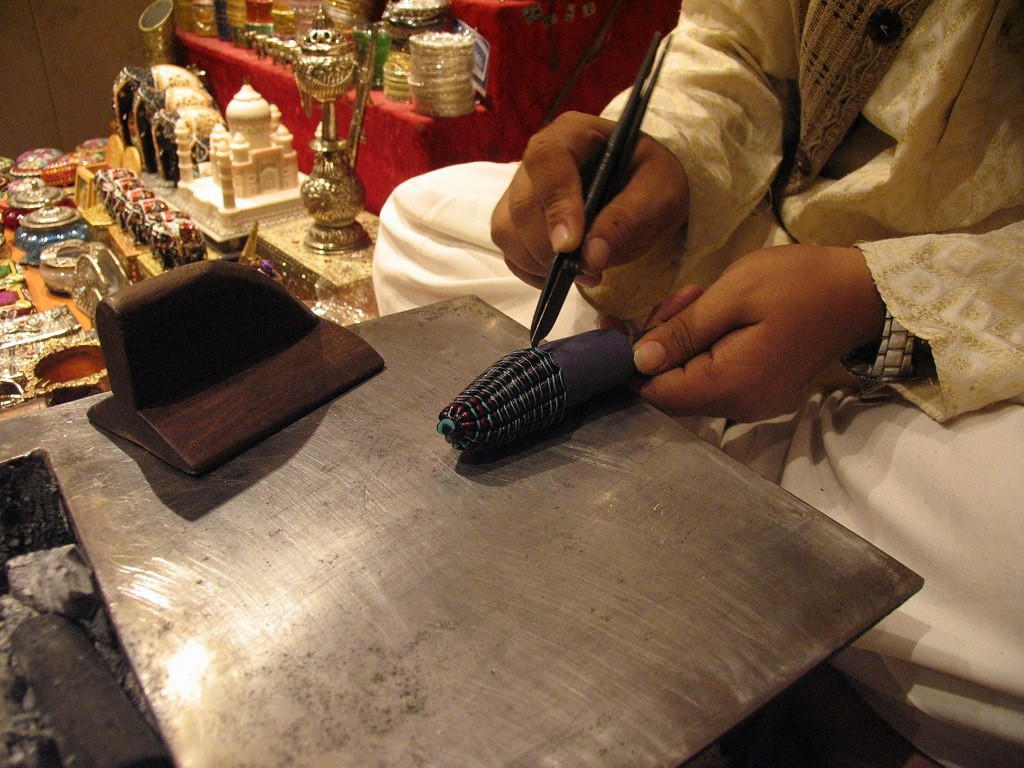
[492,0,1024,768]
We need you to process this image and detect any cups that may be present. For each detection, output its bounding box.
[353,24,395,89]
[254,35,300,73]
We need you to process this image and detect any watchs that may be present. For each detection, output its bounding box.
[840,309,915,383]
